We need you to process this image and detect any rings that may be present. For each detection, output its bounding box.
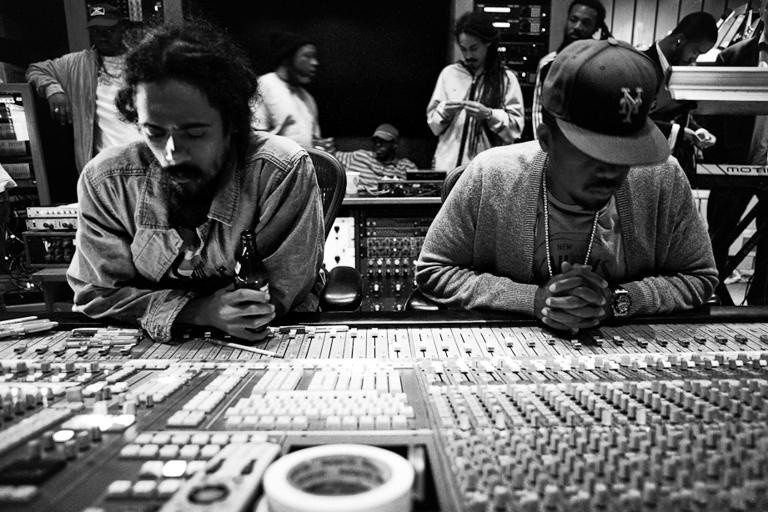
[54,107,59,113]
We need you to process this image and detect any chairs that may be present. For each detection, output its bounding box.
[302,145,364,311]
[404,164,468,316]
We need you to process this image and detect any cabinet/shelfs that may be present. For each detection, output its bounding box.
[32,265,105,328]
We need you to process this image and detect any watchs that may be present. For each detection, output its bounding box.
[610,287,633,318]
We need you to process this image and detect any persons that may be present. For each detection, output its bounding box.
[531,1,615,140]
[714,0,768,66]
[248,28,338,153]
[640,11,717,188]
[26,0,146,174]
[414,37,721,332]
[335,122,420,194]
[65,15,327,344]
[425,10,526,174]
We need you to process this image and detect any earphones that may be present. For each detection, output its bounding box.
[676,39,681,47]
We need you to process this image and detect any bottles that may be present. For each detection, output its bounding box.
[233,230,272,335]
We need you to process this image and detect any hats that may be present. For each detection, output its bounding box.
[538,35,672,169]
[369,122,400,143]
[84,3,124,29]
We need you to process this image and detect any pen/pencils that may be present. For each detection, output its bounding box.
[205,337,284,358]
